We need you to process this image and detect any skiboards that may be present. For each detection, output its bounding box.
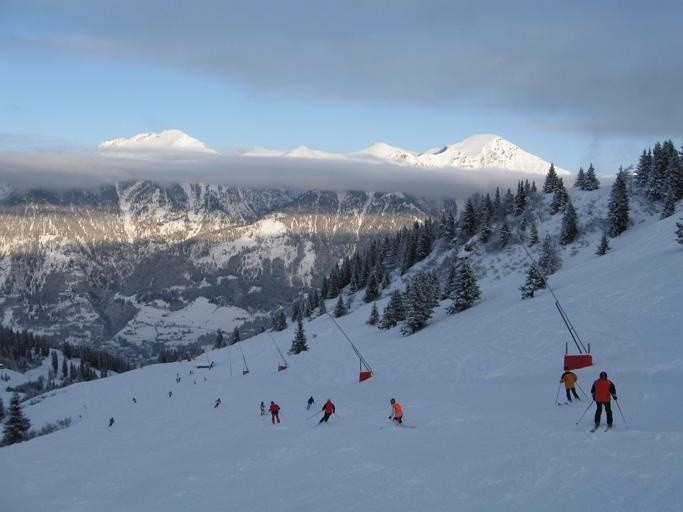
[397,425,415,429]
[590,426,611,432]
[557,402,568,405]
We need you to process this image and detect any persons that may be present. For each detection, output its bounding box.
[109,417,114,427]
[307,396,335,423]
[560,366,617,426]
[260,401,280,424]
[214,398,222,408]
[389,399,403,424]
